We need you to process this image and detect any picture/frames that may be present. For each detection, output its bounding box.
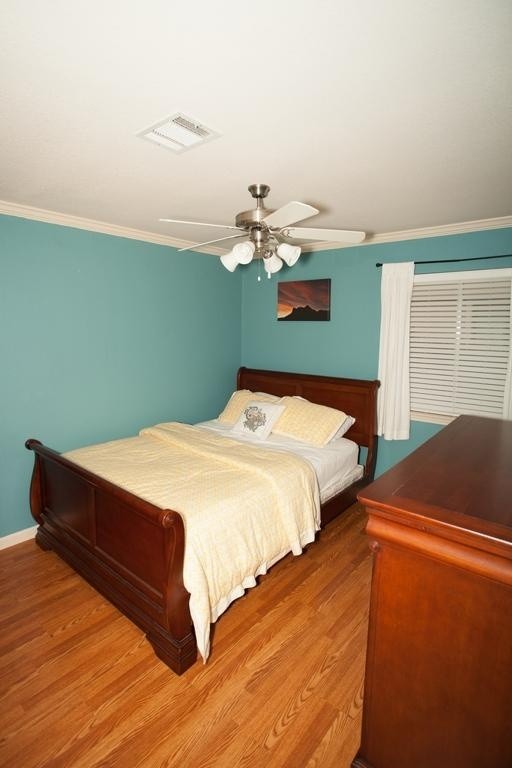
[276,278,331,322]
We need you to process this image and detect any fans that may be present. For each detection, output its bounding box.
[157,184,366,252]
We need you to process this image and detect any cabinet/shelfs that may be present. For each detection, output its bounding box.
[353,415,512,767]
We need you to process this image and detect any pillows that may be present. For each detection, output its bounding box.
[270,396,346,448]
[290,395,354,444]
[216,389,264,429]
[230,399,284,440]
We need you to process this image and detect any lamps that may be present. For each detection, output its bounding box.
[220,232,301,282]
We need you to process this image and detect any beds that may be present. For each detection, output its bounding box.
[25,366,381,676]
